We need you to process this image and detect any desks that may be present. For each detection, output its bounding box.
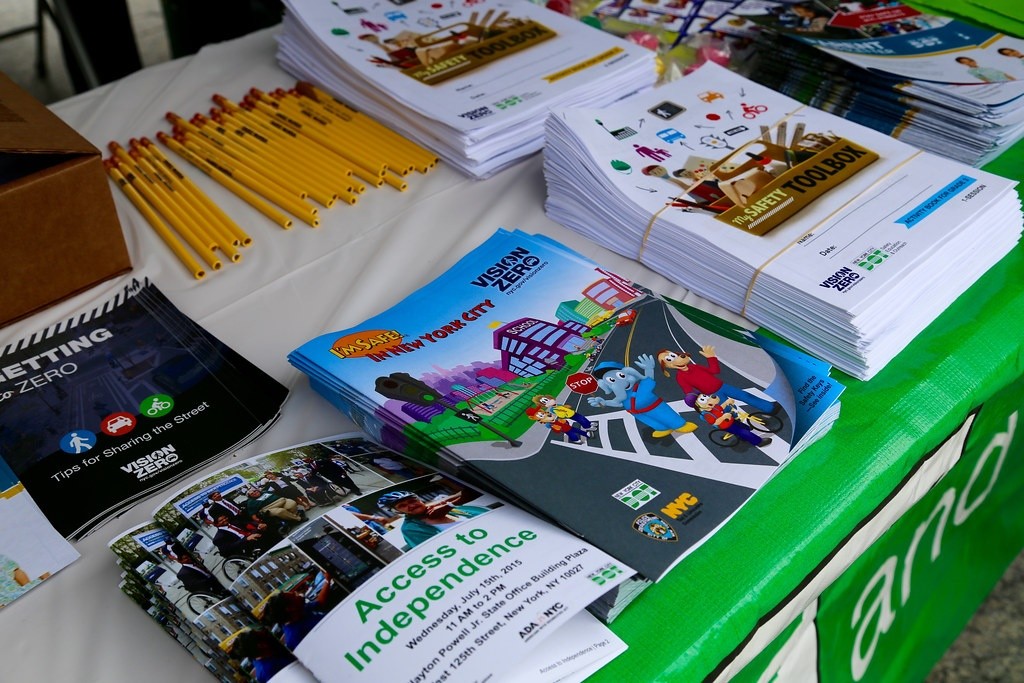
[0,22,1024,683]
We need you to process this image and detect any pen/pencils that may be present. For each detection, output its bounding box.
[101,79,442,282]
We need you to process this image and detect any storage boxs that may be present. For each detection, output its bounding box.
[0,66,133,329]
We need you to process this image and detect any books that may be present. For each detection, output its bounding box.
[0,0,1024,683]
